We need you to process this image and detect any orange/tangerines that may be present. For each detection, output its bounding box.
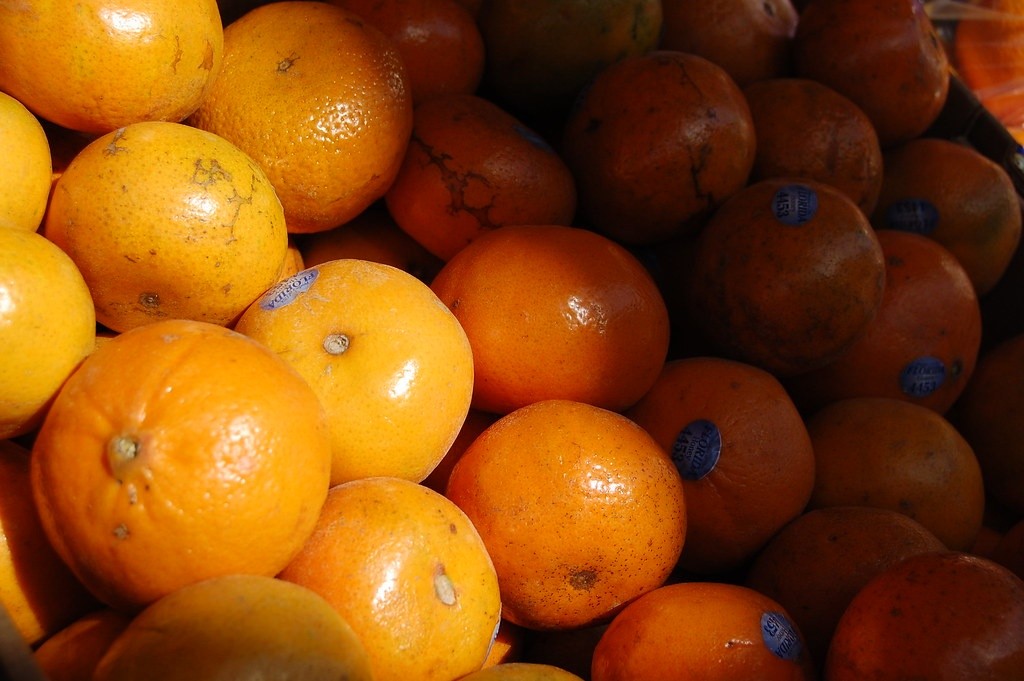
[0,0,1024,681]
